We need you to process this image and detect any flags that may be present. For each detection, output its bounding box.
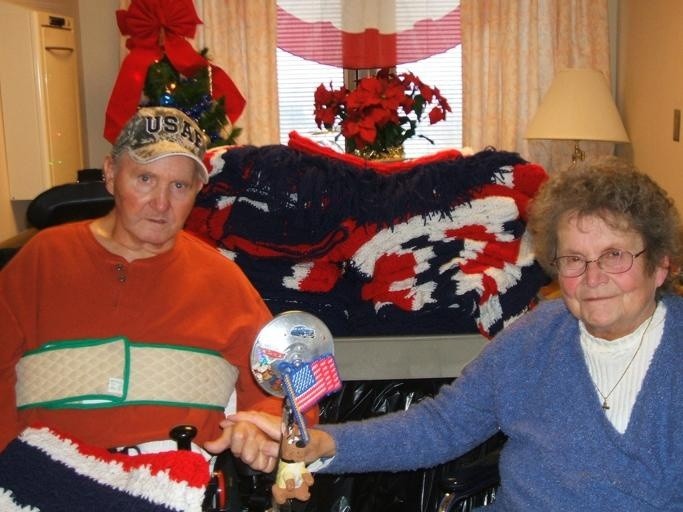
[283,354,343,410]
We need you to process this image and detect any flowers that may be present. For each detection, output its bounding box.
[312,65,453,157]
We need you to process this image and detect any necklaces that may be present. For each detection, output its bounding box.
[588,312,655,412]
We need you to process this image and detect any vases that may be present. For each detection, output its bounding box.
[347,138,404,162]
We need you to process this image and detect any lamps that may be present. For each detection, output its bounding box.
[523,67,631,165]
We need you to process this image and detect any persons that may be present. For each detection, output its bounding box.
[216,152,682,511]
[0,105,318,511]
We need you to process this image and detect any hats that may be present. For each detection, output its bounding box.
[111,105,209,184]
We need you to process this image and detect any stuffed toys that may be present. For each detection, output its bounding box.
[271,421,315,505]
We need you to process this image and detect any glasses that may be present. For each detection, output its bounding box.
[550,247,647,279]
